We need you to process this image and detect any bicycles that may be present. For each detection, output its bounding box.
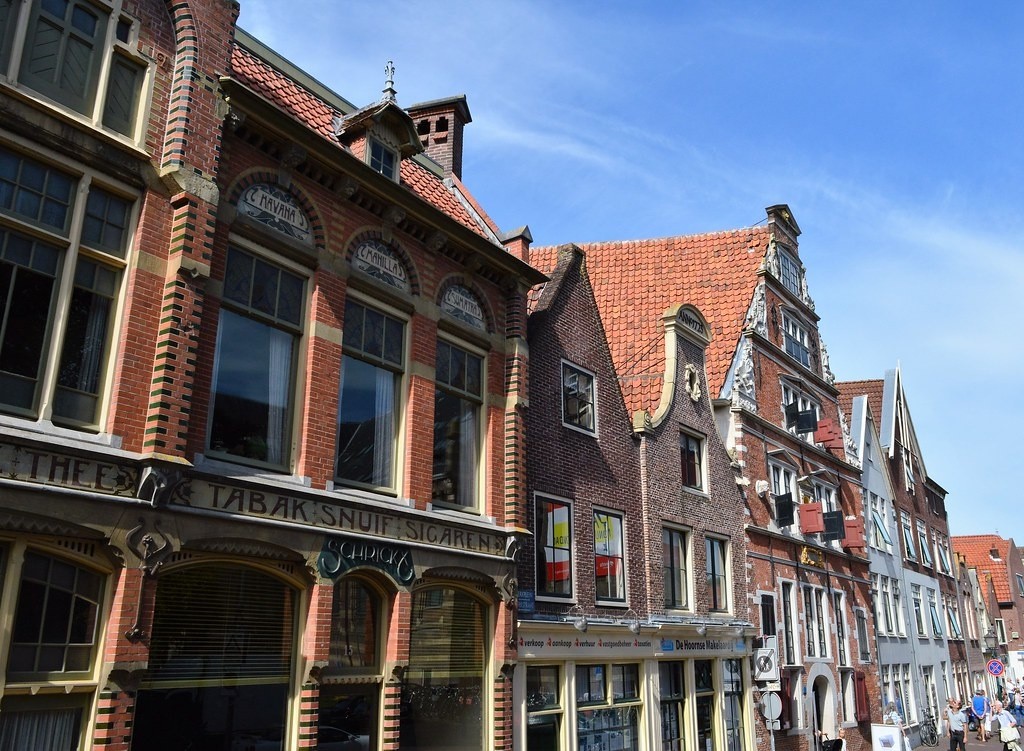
[919,708,940,747]
[400,682,481,720]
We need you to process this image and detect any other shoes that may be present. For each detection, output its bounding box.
[1017,725,1021,729]
[994,730,999,733]
[986,731,990,738]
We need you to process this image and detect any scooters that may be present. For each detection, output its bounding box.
[965,701,976,732]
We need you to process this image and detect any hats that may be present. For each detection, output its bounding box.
[975,689,981,694]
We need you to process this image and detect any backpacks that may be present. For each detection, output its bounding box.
[885,711,895,725]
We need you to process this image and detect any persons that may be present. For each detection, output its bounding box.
[970,690,987,741]
[882,701,907,736]
[989,701,1018,751]
[1005,676,1024,715]
[981,690,991,738]
[836,728,847,751]
[1011,688,1024,728]
[943,698,967,751]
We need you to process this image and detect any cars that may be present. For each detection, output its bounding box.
[232,725,370,750]
[319,693,348,708]
[330,694,373,720]
